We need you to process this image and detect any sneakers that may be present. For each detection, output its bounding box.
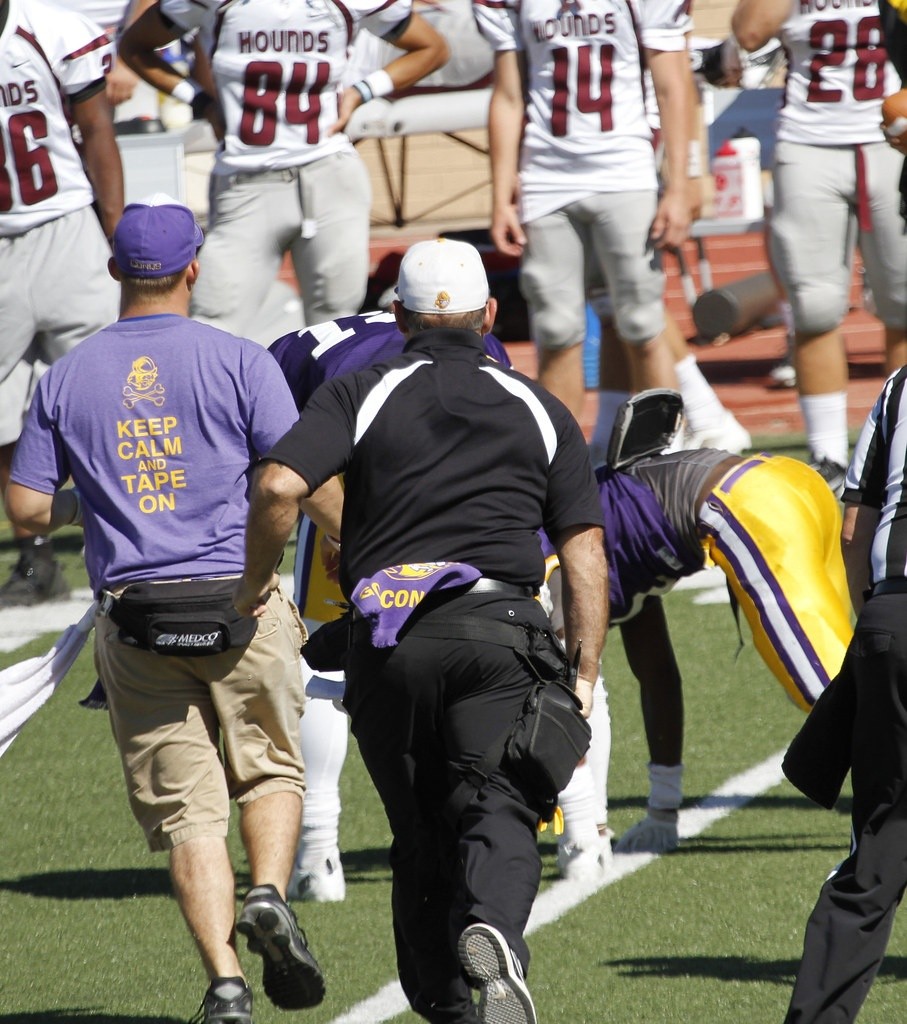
[764,358,798,389]
[236,884,324,1011]
[805,449,848,498]
[286,852,346,902]
[661,414,752,456]
[188,976,253,1024]
[457,923,536,1024]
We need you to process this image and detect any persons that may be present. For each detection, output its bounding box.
[782,364,907,1024]
[550,448,855,853]
[0,0,193,607]
[263,314,612,903]
[114,0,451,334]
[4,192,345,1024]
[235,238,610,1024]
[471,0,752,453]
[730,0,907,492]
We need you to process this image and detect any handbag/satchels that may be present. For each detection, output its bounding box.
[93,577,259,657]
[504,678,592,802]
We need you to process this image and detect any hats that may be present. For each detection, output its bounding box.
[111,201,204,277]
[395,238,490,314]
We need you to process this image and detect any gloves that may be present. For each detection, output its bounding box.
[612,814,680,853]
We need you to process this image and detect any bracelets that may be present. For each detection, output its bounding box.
[354,69,393,101]
[69,490,82,526]
[326,535,340,551]
[172,79,214,114]
[688,141,703,176]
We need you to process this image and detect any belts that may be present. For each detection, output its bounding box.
[450,578,530,596]
[104,117,167,136]
[872,578,907,596]
[720,452,772,494]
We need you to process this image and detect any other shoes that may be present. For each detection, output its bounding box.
[0,551,71,608]
